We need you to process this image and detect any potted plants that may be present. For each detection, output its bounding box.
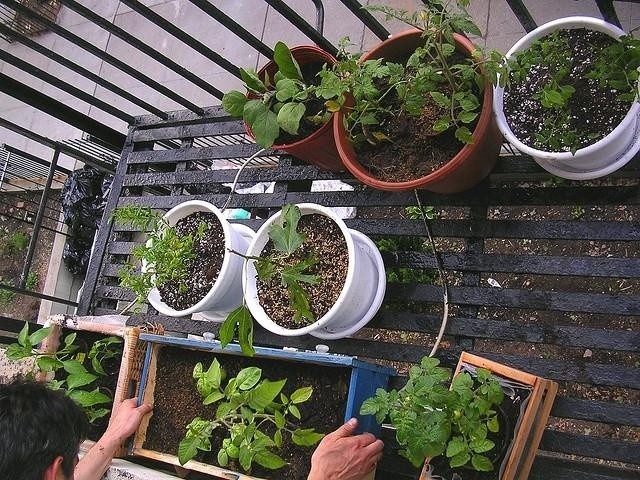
[314,0,502,194]
[128,333,395,480]
[493,16,640,180]
[107,200,256,323]
[4,315,163,456]
[219,200,386,356]
[222,41,343,172]
[359,351,558,480]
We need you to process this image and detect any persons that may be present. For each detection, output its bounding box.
[0,378,385,480]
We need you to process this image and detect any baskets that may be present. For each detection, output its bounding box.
[32,315,165,457]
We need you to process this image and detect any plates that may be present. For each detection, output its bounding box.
[198,224,255,322]
[533,104,640,181]
[308,229,386,339]
